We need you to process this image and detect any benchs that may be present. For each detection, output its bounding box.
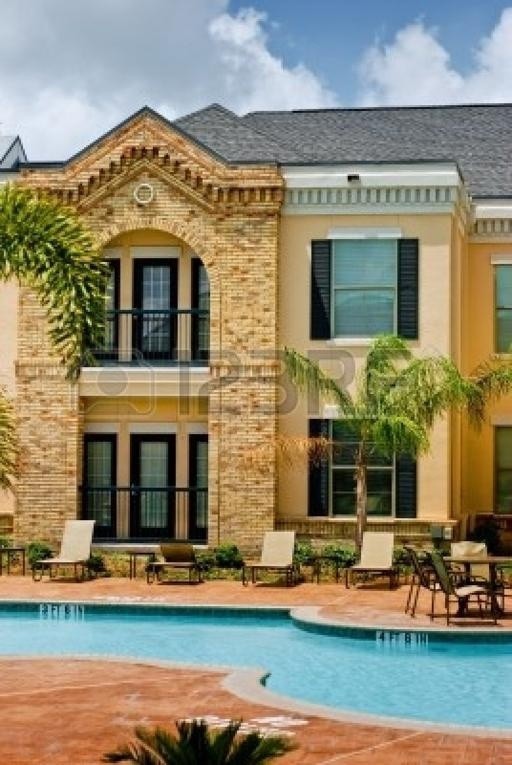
[145,562,201,585]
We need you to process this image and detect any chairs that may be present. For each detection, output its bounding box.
[430,547,504,626]
[344,531,399,588]
[451,541,503,615]
[403,546,461,619]
[36,520,97,583]
[241,531,299,586]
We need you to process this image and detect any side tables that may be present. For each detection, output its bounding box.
[127,550,155,580]
[0,546,26,576]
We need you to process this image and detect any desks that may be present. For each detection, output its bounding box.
[442,557,512,617]
